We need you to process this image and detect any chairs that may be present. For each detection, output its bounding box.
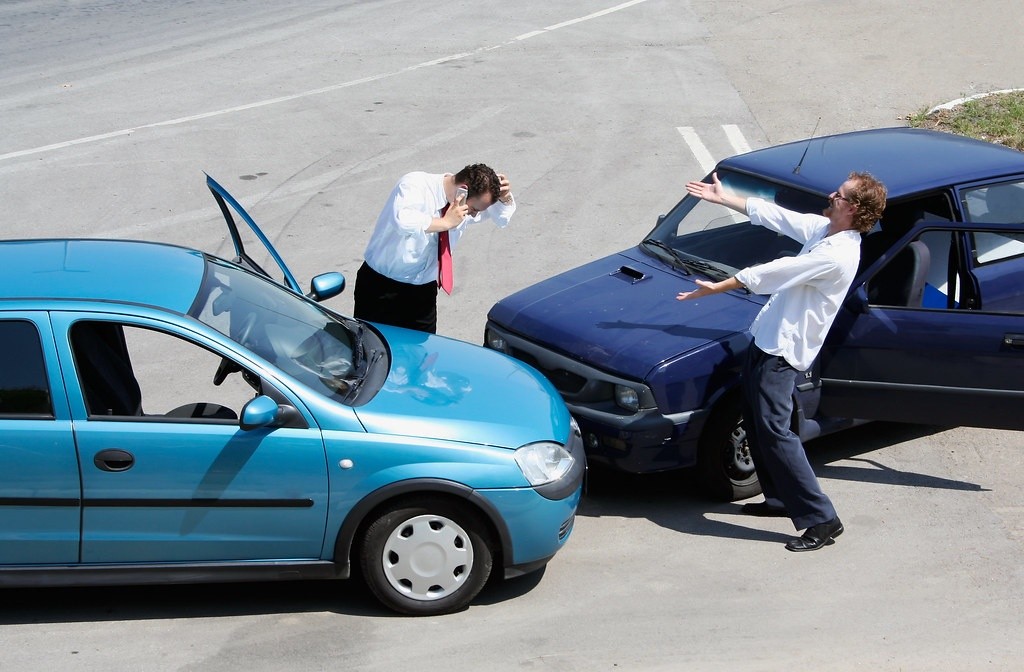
[865,198,930,307]
[976,184,1024,243]
[83,340,237,423]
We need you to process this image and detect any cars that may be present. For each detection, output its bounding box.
[482,128,1023,501]
[0,169,589,617]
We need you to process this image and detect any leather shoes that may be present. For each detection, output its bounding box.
[741,501,790,517]
[786,516,844,552]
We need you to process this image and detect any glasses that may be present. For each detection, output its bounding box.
[833,191,853,203]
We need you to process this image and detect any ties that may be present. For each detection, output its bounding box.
[441,202,453,295]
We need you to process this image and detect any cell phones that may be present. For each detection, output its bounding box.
[455,187,468,207]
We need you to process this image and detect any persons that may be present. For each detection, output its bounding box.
[352,162,518,335]
[675,169,888,552]
